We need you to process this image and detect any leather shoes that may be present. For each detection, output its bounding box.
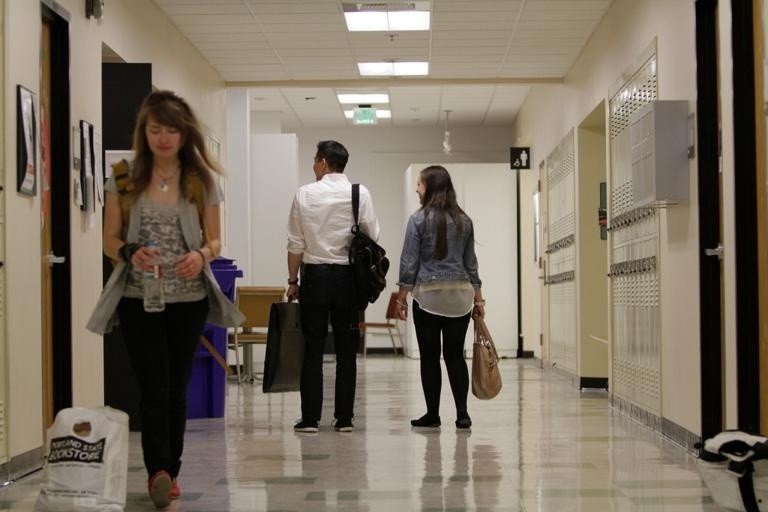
[455,418,471,428]
[411,414,441,427]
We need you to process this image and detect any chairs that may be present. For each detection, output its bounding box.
[229,286,288,384]
[361,289,407,358]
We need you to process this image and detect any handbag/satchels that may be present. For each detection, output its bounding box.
[472,316,502,399]
[262,295,304,393]
[349,233,390,304]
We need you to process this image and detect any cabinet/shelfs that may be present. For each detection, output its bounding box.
[627,100,691,204]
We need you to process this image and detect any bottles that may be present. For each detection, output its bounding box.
[141,239,164,313]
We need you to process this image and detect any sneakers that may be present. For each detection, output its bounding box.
[294,420,319,432]
[148,470,180,508]
[334,420,354,432]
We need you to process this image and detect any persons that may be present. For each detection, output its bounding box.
[84,90,247,510]
[395,164,485,433]
[285,139,380,433]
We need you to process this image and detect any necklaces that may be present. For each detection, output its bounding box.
[151,166,181,192]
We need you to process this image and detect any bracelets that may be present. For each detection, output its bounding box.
[195,248,207,272]
[396,299,407,309]
[473,299,486,307]
[116,241,141,262]
[287,277,299,286]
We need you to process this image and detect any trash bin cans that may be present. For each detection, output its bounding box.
[185,256,243,419]
